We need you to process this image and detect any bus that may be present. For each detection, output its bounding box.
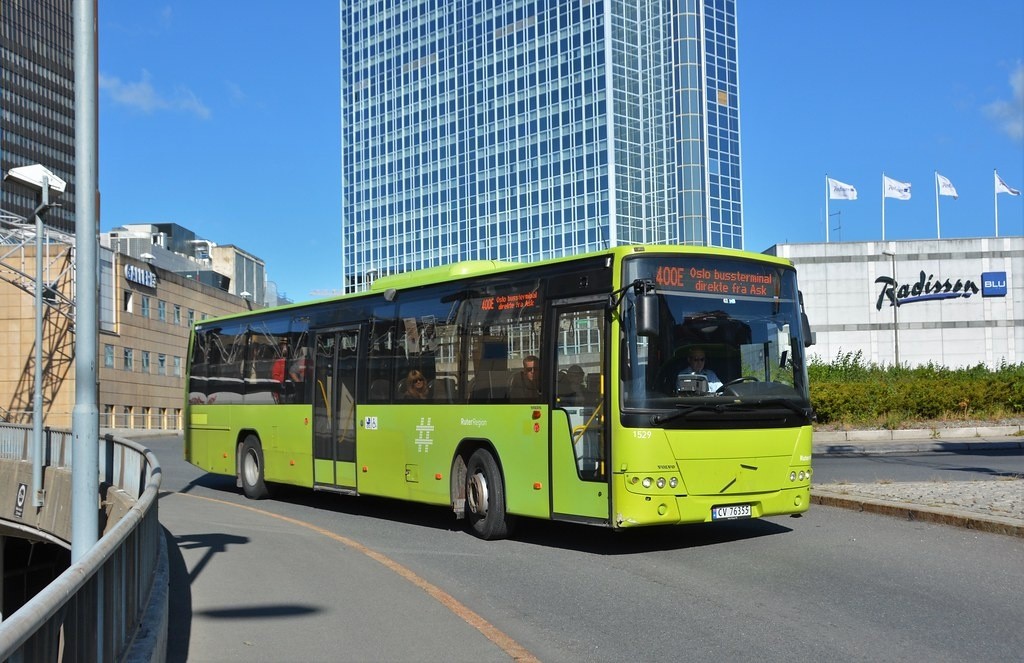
[179,243,818,540]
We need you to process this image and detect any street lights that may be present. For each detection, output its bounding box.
[882,250,900,367]
[4,162,66,507]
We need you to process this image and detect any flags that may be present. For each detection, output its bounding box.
[828,177,858,200]
[937,173,958,200]
[996,173,1021,197]
[883,176,912,200]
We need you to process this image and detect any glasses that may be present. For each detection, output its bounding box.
[526,367,540,372]
[412,377,424,383]
[690,356,705,362]
[568,372,584,376]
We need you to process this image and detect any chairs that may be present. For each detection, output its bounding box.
[428,378,444,399]
[558,367,568,381]
[395,377,408,399]
[373,377,393,401]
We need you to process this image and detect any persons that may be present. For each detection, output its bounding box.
[400,369,432,400]
[272,343,288,405]
[240,342,261,379]
[567,365,595,406]
[509,355,540,403]
[289,357,314,382]
[675,347,723,397]
